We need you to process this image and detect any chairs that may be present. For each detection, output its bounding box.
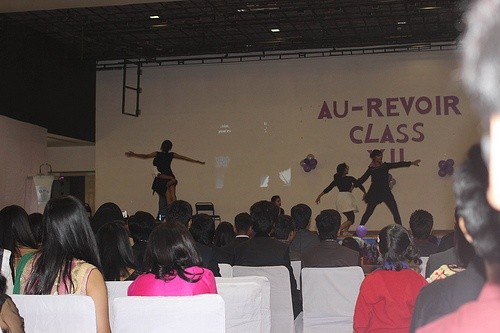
[104,281,134,331]
[9,295,97,333]
[195,201,221,225]
[231,265,303,333]
[111,293,225,333]
[214,276,271,333]
[302,266,365,333]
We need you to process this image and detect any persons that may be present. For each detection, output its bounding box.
[126,139,205,223]
[229,201,301,321]
[271,195,284,215]
[411,209,500,333]
[13,194,111,333]
[0,199,359,333]
[454,0,500,209]
[315,162,367,237]
[351,149,421,237]
[408,209,438,257]
[128,223,217,297]
[302,210,360,268]
[416,143,500,333]
[353,224,430,333]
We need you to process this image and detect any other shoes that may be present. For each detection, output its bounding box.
[343,231,354,236]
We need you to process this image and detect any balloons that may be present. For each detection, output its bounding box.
[356,225,368,238]
[438,159,454,177]
[300,154,317,172]
